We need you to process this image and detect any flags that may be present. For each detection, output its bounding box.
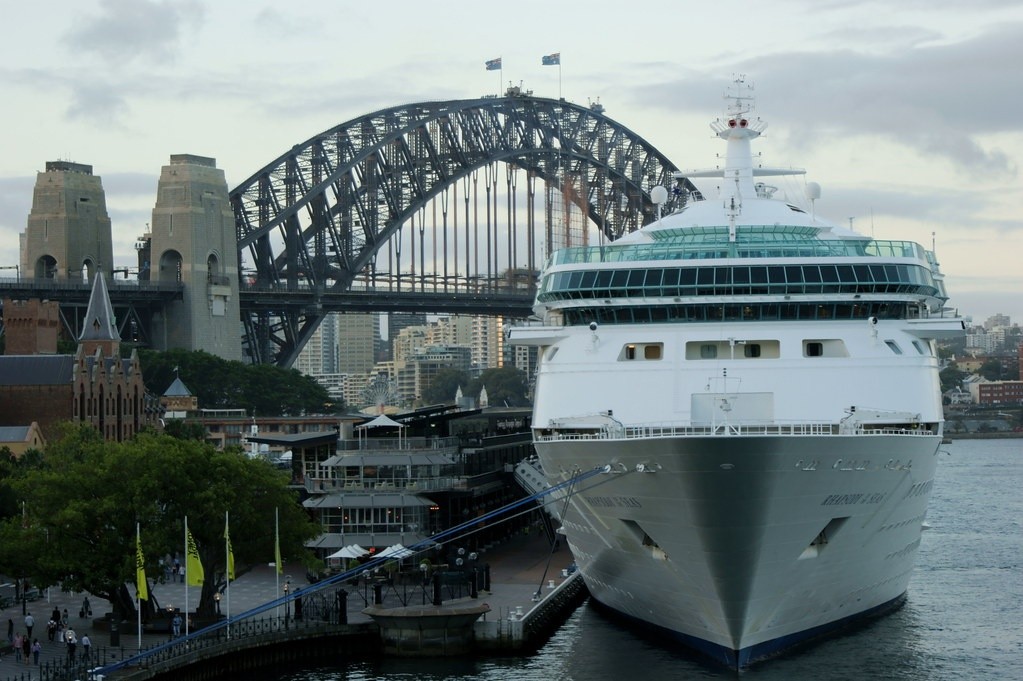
[275,534,283,574]
[486,58,501,71]
[136,535,148,601]
[224,526,235,580]
[542,53,560,65]
[187,527,204,587]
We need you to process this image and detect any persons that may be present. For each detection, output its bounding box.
[83,597,91,619]
[82,634,91,662]
[65,627,78,653]
[188,615,194,634]
[45,606,68,642]
[537,518,545,536]
[14,632,41,665]
[24,612,35,638]
[522,517,534,536]
[7,619,13,641]
[172,613,182,637]
[158,551,186,583]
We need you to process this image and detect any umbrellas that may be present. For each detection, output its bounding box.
[326,543,370,579]
[370,543,415,578]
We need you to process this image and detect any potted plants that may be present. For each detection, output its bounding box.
[301,550,325,585]
[385,558,399,586]
[349,560,360,586]
[420,558,434,587]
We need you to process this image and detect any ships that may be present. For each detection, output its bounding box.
[506,68,966,678]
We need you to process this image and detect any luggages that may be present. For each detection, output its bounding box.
[80,610,84,617]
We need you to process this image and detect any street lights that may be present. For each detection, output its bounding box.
[420,563,429,604]
[282,584,289,628]
[213,592,222,641]
[455,558,463,598]
[165,603,174,655]
[469,552,477,598]
[458,547,466,584]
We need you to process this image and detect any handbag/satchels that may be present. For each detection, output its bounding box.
[88,608,92,615]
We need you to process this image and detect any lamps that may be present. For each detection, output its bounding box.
[868,316,878,339]
[590,321,600,350]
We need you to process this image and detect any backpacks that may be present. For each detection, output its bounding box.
[48,620,55,629]
